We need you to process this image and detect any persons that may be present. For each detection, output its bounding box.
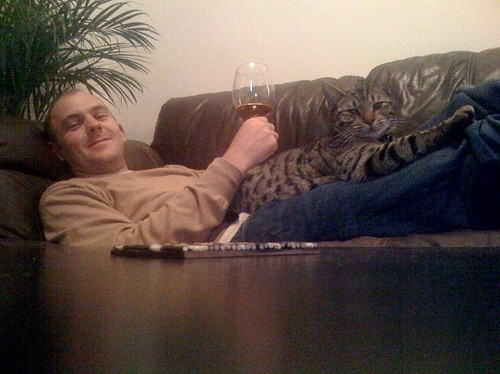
[39,80,499,245]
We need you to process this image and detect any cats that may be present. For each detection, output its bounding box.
[224,68,475,221]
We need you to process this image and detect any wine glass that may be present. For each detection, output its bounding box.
[231,63,274,121]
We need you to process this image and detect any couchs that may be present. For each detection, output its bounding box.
[0,46,500,249]
